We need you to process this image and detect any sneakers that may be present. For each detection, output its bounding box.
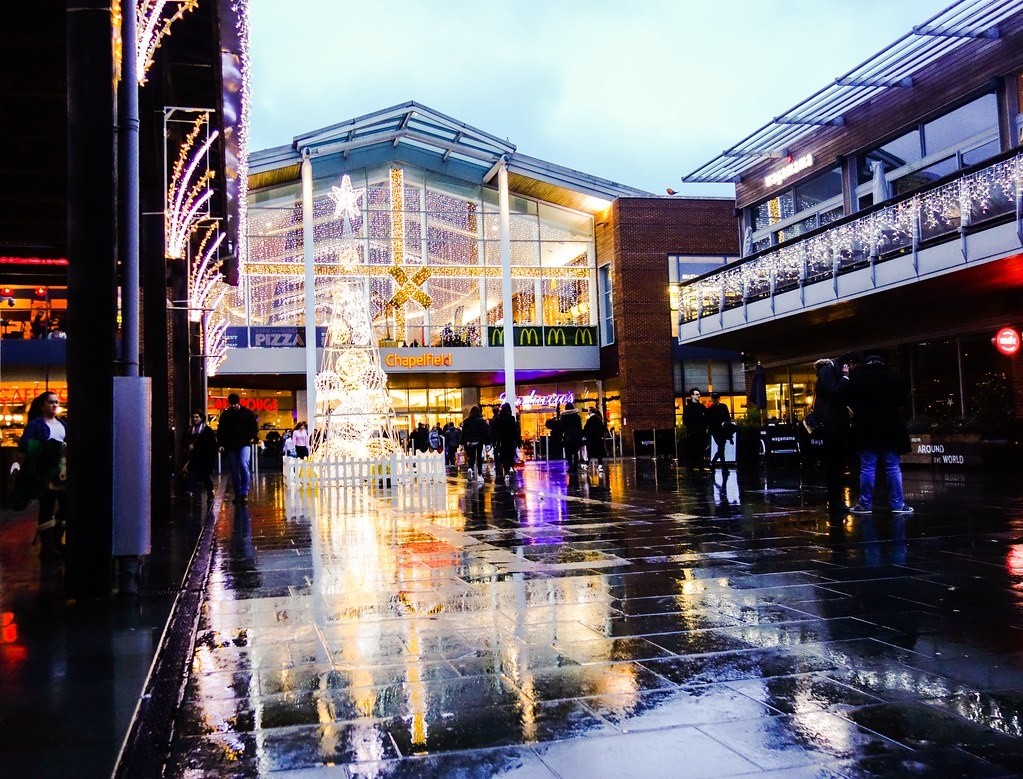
[849,505,873,513]
[892,503,914,513]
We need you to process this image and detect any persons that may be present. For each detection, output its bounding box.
[216,394,259,505]
[32,310,64,340]
[559,402,605,472]
[264,421,326,474]
[682,388,733,474]
[442,321,479,347]
[18,392,67,561]
[407,402,523,484]
[410,339,419,347]
[811,352,917,515]
[179,410,217,504]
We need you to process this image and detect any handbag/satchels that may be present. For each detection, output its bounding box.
[175,457,191,475]
[797,413,834,457]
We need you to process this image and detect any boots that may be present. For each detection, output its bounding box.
[31,515,66,578]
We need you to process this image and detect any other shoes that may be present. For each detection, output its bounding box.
[206,492,215,502]
[581,464,588,470]
[597,465,603,471]
[566,464,578,472]
[467,467,473,482]
[722,471,730,474]
[233,494,248,505]
[477,476,484,482]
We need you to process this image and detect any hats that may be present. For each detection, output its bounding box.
[711,392,720,399]
